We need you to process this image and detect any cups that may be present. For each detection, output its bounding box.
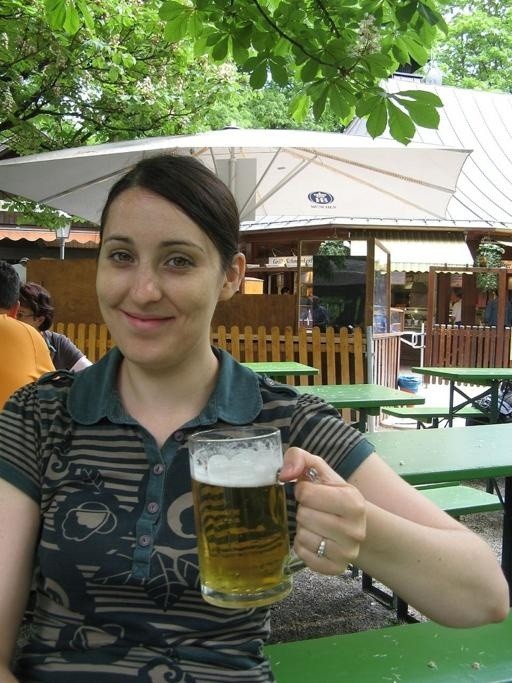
[189,424,323,605]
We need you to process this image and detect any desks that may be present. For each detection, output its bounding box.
[239,361,320,386]
[361,419,512,624]
[293,382,426,434]
[411,366,512,427]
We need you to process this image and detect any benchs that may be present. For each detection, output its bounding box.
[264,604,512,683]
[381,406,492,428]
[277,485,505,579]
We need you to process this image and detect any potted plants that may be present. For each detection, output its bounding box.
[317,240,350,273]
[474,243,506,291]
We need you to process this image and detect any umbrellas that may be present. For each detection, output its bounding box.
[0,124,473,235]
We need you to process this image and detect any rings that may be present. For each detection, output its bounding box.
[316,535,326,557]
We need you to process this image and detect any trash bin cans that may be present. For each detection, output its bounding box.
[398,376,420,408]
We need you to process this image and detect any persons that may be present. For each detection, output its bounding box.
[0,147,512,681]
[281,286,290,294]
[1,259,59,408]
[485,286,512,327]
[449,287,462,327]
[17,279,94,373]
[316,287,354,324]
